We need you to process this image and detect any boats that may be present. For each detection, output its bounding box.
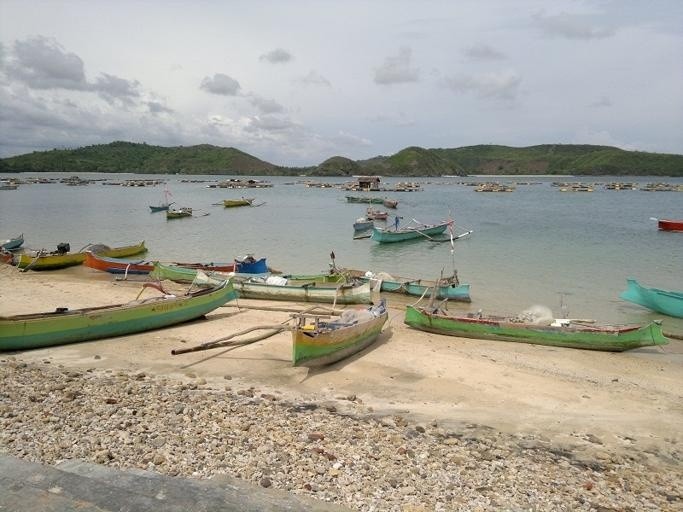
[620,278,683,320]
[148,201,175,212]
[345,195,454,245]
[224,198,255,208]
[658,218,683,231]
[165,208,192,219]
[404,286,670,354]
